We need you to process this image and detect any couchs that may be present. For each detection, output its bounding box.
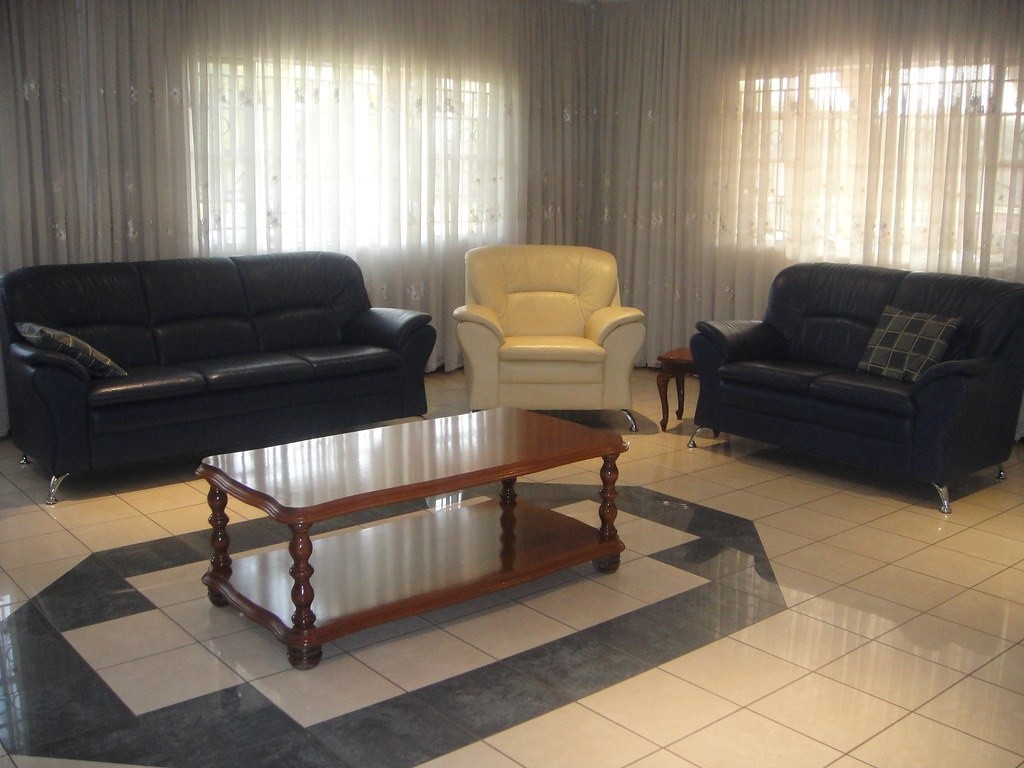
[685,262,1024,515]
[451,243,647,434]
[0,251,438,506]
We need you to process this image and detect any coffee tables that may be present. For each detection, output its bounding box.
[192,406,631,671]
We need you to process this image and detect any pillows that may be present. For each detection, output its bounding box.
[13,319,128,380]
[854,303,965,385]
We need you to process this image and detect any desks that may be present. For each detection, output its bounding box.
[654,345,722,439]
